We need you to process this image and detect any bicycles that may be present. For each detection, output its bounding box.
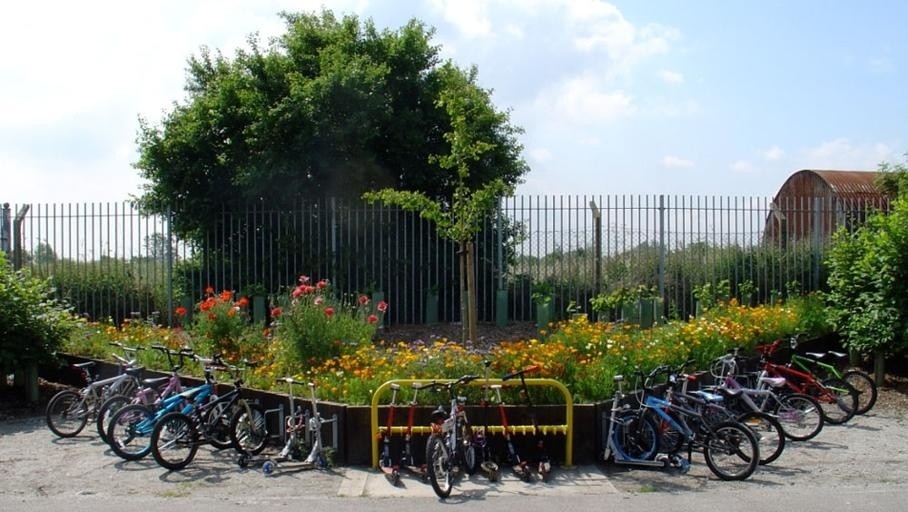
[43,342,271,478]
[408,372,485,502]
